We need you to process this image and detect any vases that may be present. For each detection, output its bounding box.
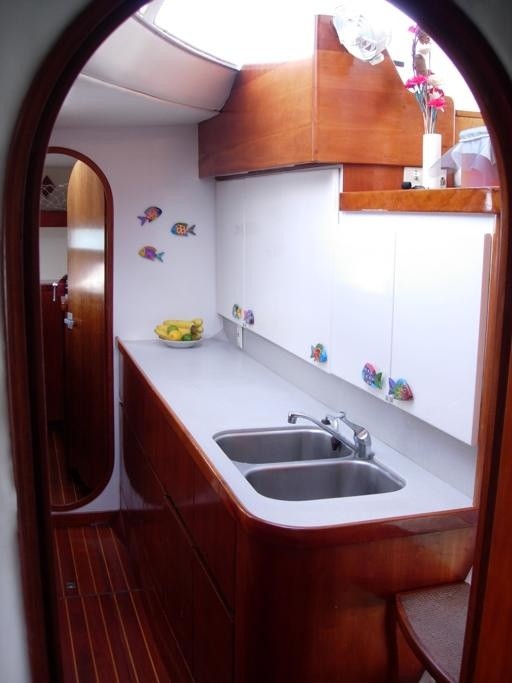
[421,133,443,189]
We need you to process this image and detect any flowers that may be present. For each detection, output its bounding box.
[403,23,449,131]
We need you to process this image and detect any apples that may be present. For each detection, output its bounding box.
[169,330,179,340]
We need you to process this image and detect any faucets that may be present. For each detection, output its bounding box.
[285,414,374,459]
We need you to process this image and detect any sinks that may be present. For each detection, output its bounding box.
[212,427,354,464]
[245,462,403,502]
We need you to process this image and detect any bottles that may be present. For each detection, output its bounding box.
[453,125,501,188]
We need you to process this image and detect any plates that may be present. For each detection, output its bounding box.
[159,337,202,348]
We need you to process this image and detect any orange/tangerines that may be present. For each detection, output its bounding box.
[167,325,178,333]
[183,335,191,341]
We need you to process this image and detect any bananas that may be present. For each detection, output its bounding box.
[155,319,202,340]
